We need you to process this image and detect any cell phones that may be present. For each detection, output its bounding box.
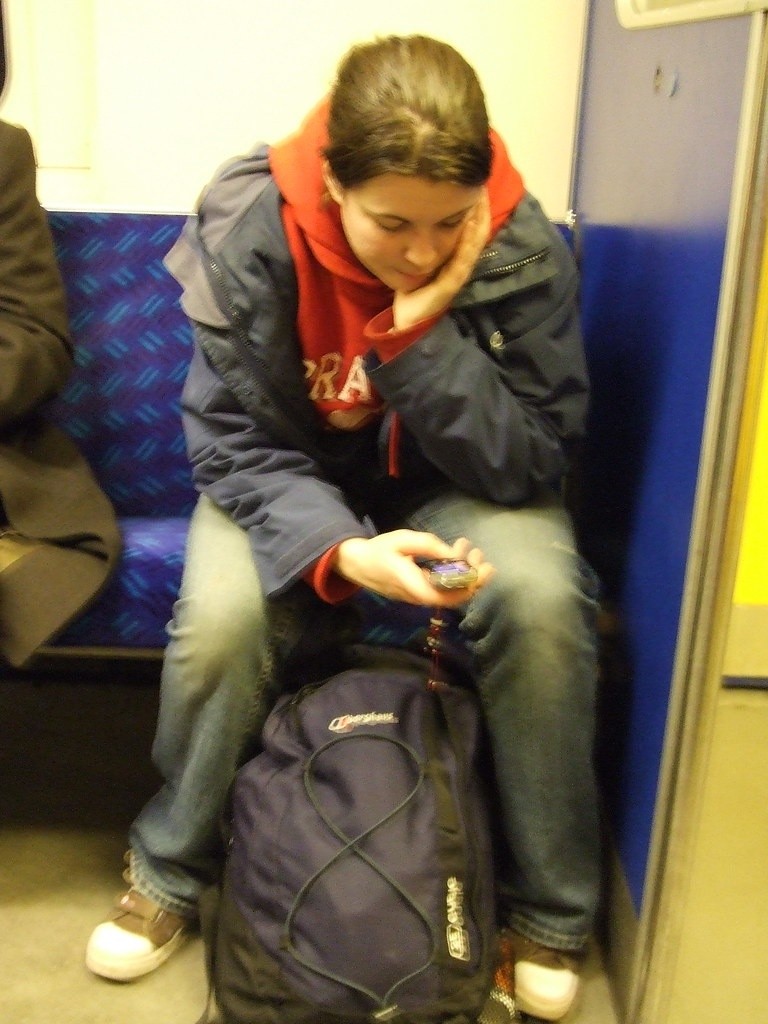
[419,556,479,591]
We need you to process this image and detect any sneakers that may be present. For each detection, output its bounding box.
[512,929,584,1021]
[85,886,198,981]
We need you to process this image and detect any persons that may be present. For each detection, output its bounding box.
[86,36,605,1020]
[0,0,122,667]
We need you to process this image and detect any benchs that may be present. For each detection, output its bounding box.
[34,212,614,661]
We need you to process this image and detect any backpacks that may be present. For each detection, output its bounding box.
[199,644,497,1024]
